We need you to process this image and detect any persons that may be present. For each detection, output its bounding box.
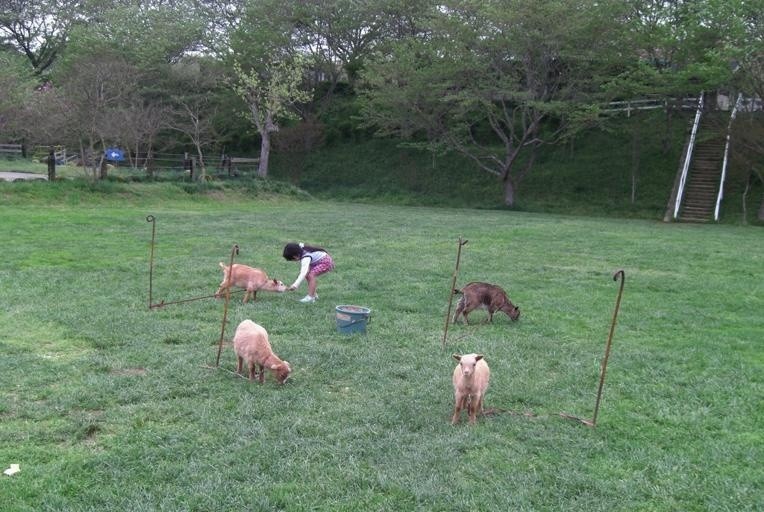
[282,242,335,303]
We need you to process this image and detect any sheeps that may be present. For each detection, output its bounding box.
[214,261,289,304]
[453,281,522,326]
[231,318,290,386]
[447,351,491,425]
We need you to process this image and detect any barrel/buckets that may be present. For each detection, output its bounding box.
[335,305,372,336]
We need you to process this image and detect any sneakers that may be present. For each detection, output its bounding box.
[298,293,319,303]
